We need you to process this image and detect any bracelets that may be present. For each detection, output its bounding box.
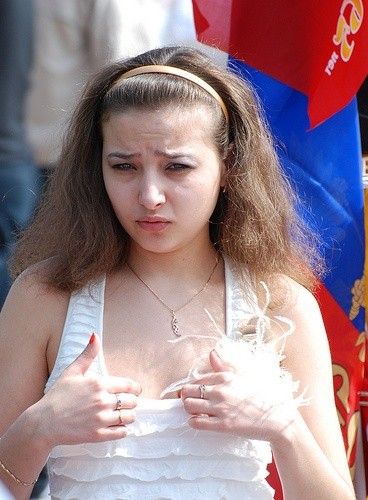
[0,460,39,487]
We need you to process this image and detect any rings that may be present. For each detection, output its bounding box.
[198,383,208,399]
[113,394,122,412]
[118,408,123,427]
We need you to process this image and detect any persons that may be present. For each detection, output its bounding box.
[0,0,229,315]
[0,46,358,500]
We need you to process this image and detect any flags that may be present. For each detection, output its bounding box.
[189,1,368,500]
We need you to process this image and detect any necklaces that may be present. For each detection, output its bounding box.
[123,249,222,338]
